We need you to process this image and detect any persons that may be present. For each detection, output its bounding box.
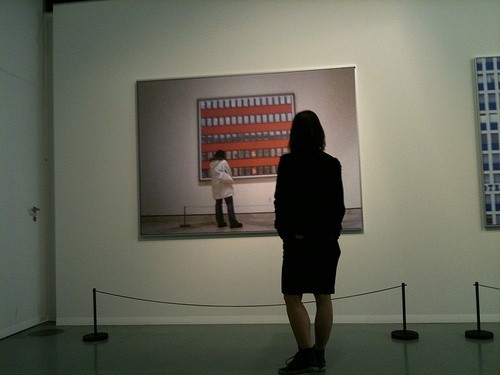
[208,151,243,228]
[273,110,346,375]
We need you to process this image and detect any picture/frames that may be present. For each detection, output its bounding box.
[136,66,363,237]
[196,93,296,181]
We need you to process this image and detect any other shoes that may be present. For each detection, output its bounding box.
[218,222,227,228]
[278,346,325,375]
[230,223,243,229]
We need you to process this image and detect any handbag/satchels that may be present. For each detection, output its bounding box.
[219,161,234,184]
